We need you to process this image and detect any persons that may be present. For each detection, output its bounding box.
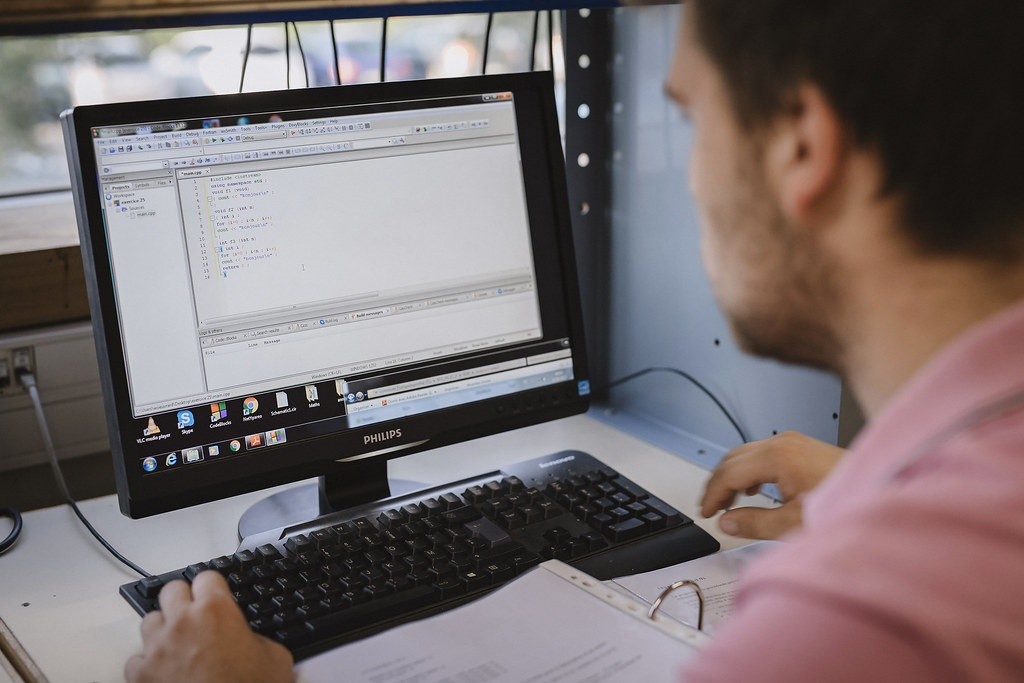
[121,0,1024,683]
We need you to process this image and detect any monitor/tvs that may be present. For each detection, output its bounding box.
[58,70,591,539]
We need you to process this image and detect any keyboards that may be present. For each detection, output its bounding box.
[119,448,723,662]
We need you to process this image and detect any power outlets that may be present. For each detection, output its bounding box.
[7,346,34,388]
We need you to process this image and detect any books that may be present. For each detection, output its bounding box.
[296,539,774,683]
